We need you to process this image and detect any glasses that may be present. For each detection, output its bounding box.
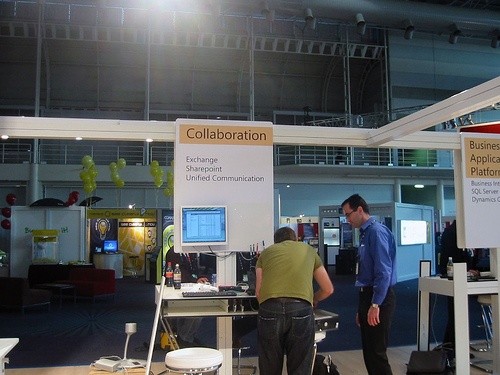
[345,208,356,220]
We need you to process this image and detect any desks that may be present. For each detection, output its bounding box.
[144,282,259,375]
[34,284,77,306]
[417,261,500,375]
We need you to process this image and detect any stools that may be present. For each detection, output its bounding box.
[165,319,257,375]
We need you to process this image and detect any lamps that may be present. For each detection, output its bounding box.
[304,7,316,30]
[447,21,461,44]
[491,28,500,49]
[403,18,415,41]
[444,113,475,130]
[356,12,366,35]
[261,2,275,22]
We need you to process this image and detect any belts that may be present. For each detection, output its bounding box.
[265,297,301,302]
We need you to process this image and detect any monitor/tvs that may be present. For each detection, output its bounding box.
[181,205,227,247]
[399,220,427,245]
[103,240,118,252]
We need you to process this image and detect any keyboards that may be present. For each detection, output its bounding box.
[477,275,495,279]
[182,290,237,297]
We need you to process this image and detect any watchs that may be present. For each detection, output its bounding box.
[372,303,379,308]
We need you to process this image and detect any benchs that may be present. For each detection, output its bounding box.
[0,276,52,315]
[54,269,115,304]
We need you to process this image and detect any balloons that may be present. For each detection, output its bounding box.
[2,208,11,218]
[150,159,174,197]
[1,219,11,229]
[80,155,98,193]
[65,192,78,207]
[110,158,126,188]
[6,193,17,205]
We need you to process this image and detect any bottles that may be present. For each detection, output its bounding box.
[447,257,454,276]
[166,262,173,287]
[173,264,181,289]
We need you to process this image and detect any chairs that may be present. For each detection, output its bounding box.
[469,295,492,353]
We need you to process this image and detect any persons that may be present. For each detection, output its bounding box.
[342,194,396,375]
[166,246,208,347]
[255,227,333,375]
[440,219,480,360]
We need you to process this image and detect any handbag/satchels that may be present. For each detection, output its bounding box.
[406,351,452,375]
